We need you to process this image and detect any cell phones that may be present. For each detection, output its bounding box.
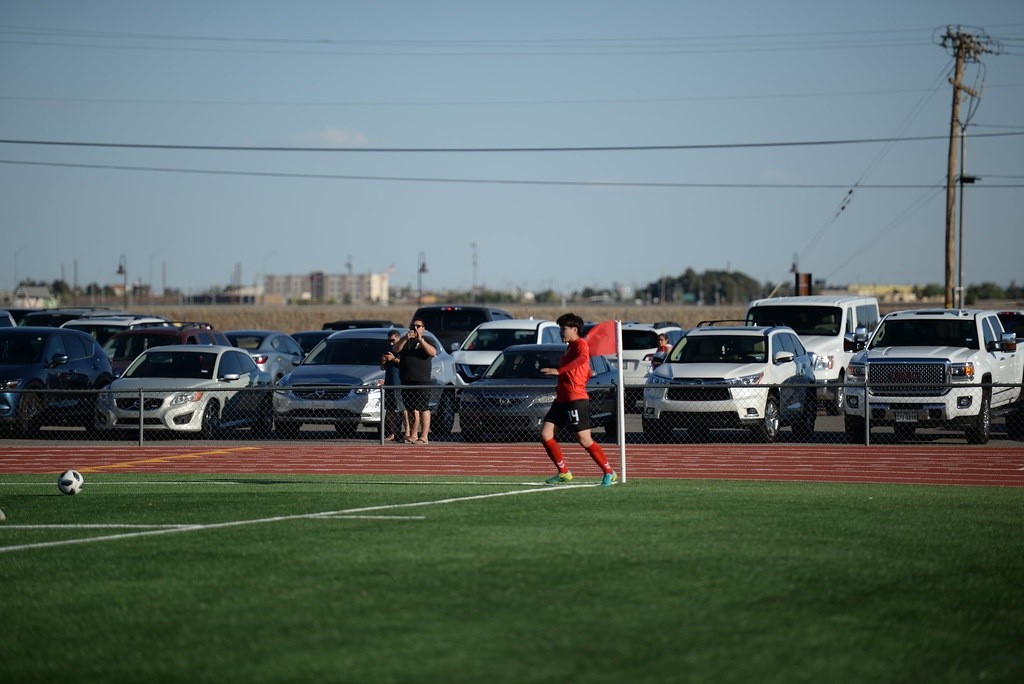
[383,352,389,355]
[410,325,415,333]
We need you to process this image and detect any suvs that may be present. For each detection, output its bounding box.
[640,317,818,442]
[584,319,726,414]
[99,318,236,385]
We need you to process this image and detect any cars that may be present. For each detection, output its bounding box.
[319,319,403,332]
[224,329,312,396]
[0,305,168,367]
[459,343,625,443]
[451,316,573,414]
[282,331,338,361]
[0,325,114,434]
[992,307,1024,341]
[271,326,459,439]
[94,344,273,440]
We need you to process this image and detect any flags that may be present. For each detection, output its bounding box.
[583,320,618,357]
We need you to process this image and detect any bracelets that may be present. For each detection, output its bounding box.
[393,357,397,362]
[418,337,424,342]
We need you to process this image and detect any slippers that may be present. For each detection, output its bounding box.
[413,438,428,444]
[403,436,419,443]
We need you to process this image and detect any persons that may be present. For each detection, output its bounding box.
[651,333,673,370]
[394,317,437,446]
[380,330,411,443]
[538,312,618,487]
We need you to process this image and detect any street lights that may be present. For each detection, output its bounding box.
[414,252,429,304]
[116,253,131,308]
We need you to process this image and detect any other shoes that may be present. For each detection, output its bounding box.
[398,436,410,443]
[385,435,396,441]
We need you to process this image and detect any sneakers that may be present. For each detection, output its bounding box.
[546,470,573,484]
[601,470,618,486]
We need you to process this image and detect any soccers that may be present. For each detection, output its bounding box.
[57,469,84,495]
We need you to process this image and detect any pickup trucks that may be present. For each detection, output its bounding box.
[842,307,1024,442]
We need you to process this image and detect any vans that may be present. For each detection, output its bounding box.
[408,303,516,359]
[743,295,882,413]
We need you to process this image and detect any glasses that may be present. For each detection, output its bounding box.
[387,338,398,342]
[411,324,423,328]
[658,339,665,342]
